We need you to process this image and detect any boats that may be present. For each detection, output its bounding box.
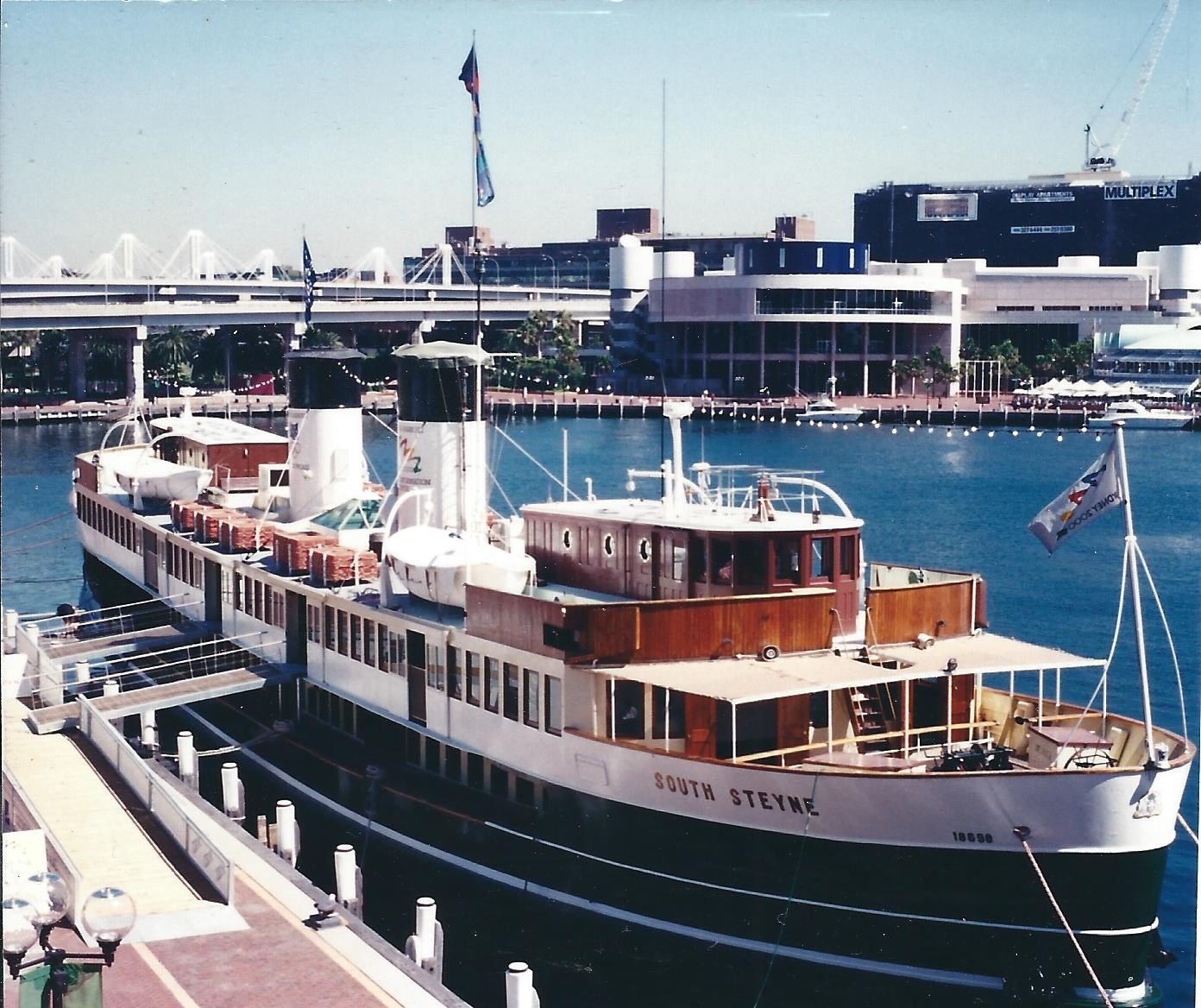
[795,398,864,424]
[73,341,1197,1008]
[1086,401,1194,429]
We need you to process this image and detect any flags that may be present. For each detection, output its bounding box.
[302,239,318,328]
[1027,439,1122,558]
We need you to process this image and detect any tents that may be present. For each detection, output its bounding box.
[1005,372,1173,402]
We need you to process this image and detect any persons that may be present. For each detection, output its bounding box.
[1012,394,1106,411]
[700,550,801,587]
